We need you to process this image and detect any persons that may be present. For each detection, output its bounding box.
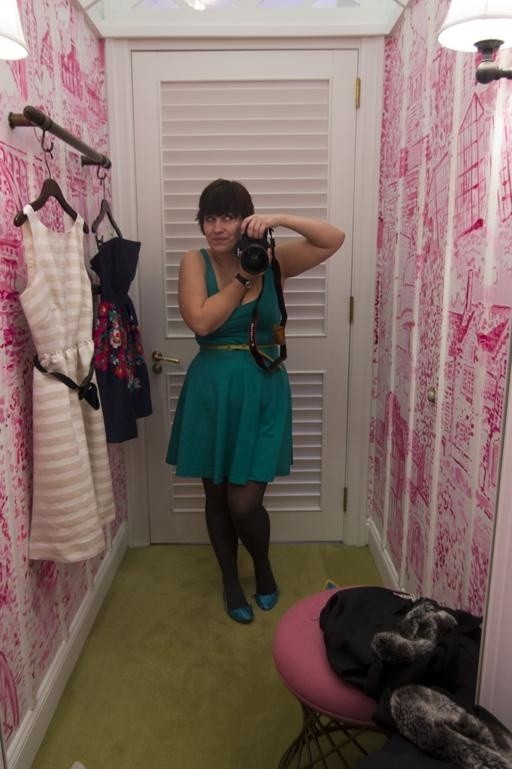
[164,176,347,625]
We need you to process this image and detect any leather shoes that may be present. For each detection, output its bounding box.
[255,587,279,612]
[222,586,254,624]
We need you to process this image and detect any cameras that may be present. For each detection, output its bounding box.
[236,224,269,274]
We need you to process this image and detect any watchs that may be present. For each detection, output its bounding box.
[232,274,254,290]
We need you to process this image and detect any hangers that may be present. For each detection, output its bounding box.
[90,177,125,238]
[14,149,90,236]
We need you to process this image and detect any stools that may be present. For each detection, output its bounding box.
[275,583,390,768]
